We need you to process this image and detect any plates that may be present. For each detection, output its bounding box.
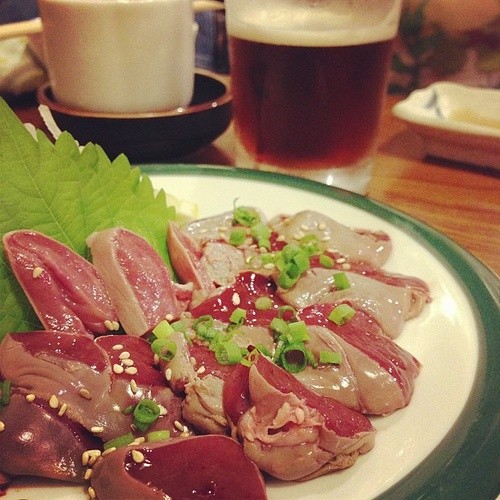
[0,164,500,498]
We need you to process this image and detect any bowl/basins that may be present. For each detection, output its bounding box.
[37,68,232,163]
[392,81,500,173]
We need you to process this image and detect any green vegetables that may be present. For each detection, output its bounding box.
[0,92,192,339]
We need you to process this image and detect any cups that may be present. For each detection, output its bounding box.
[37,0,197,112]
[223,2,403,197]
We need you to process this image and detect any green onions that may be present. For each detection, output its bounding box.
[102,205,357,450]
